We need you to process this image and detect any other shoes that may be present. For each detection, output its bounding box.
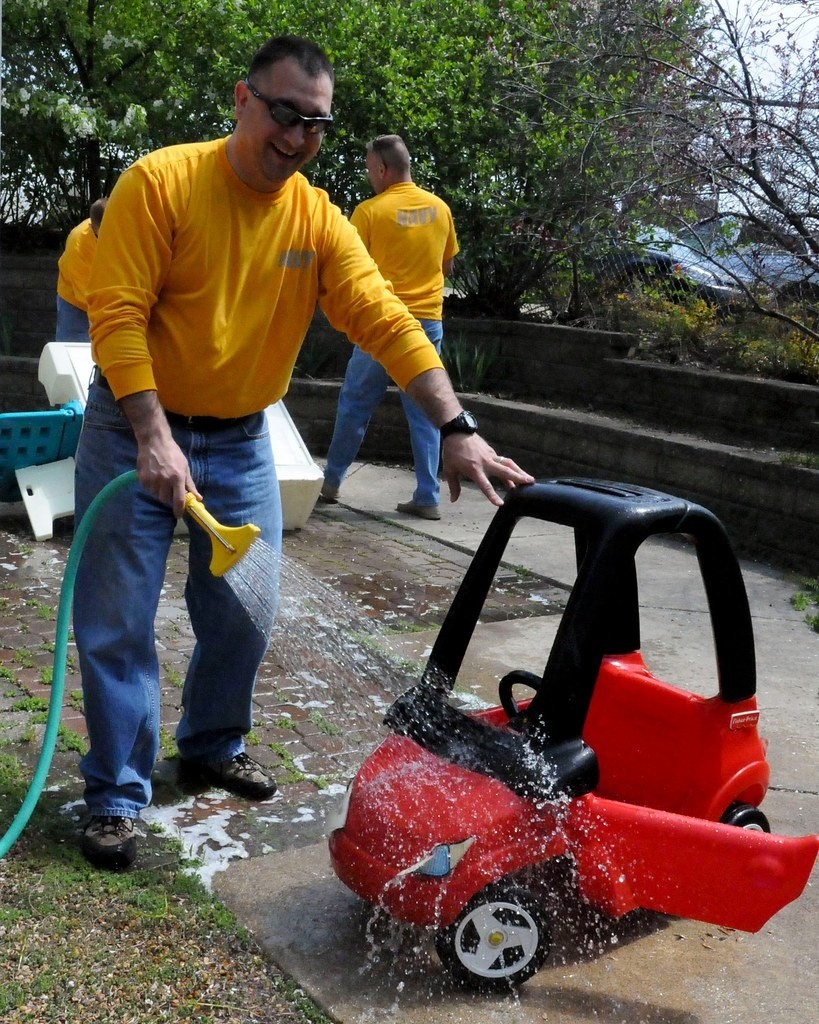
[319,491,340,503]
[398,500,441,519]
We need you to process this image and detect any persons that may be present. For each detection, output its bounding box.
[319,135,460,520]
[56,198,108,343]
[75,35,534,868]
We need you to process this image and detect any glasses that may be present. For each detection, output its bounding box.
[372,133,386,171]
[245,77,334,133]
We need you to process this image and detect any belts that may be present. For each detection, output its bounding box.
[93,368,249,430]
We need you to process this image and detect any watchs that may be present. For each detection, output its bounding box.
[440,410,478,439]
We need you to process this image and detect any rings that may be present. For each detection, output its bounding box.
[495,456,505,463]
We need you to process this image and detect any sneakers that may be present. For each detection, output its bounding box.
[179,749,277,798]
[84,813,138,867]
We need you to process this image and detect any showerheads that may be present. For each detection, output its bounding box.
[167,483,262,573]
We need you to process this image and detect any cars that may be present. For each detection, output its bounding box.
[566,208,819,310]
[324,474,819,993]
